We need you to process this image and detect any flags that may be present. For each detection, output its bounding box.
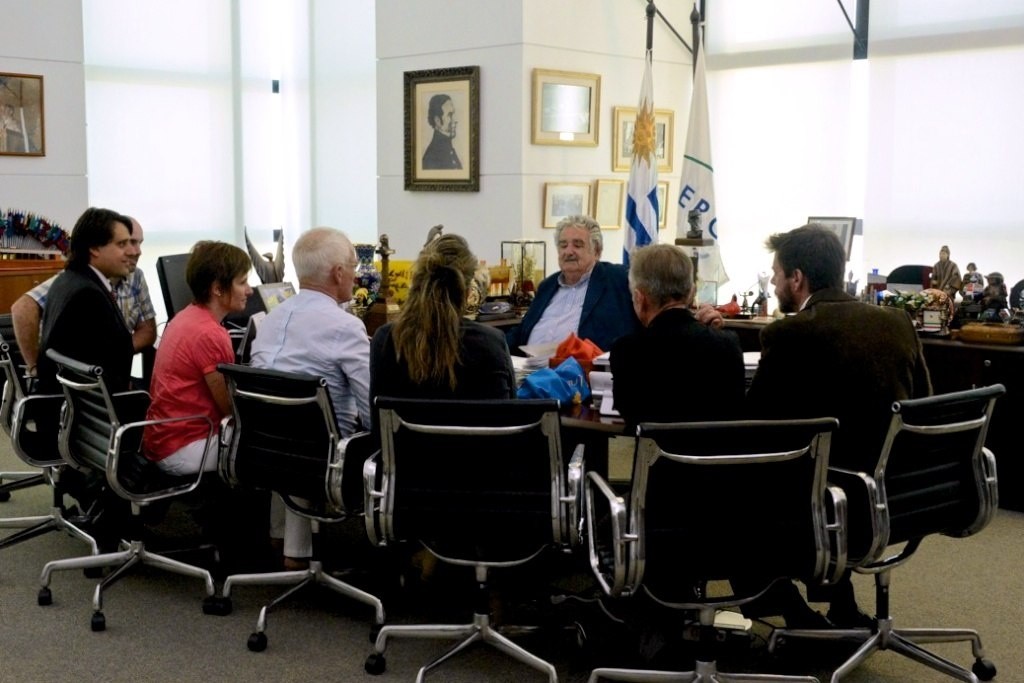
[623,50,660,270]
[673,23,731,289]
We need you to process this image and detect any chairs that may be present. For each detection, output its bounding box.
[0,255,1001,683]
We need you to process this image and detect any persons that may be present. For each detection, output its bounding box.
[370,234,518,582]
[249,225,369,513]
[140,239,314,571]
[609,243,749,436]
[932,245,1008,323]
[8,206,158,460]
[507,214,647,358]
[421,93,465,170]
[727,224,936,633]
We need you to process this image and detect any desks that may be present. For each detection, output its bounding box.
[126,320,755,438]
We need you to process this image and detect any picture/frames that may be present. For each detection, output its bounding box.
[657,180,669,227]
[0,73,47,155]
[797,217,860,261]
[613,106,677,174]
[532,65,600,148]
[595,177,622,230]
[403,66,478,192]
[542,182,588,228]
